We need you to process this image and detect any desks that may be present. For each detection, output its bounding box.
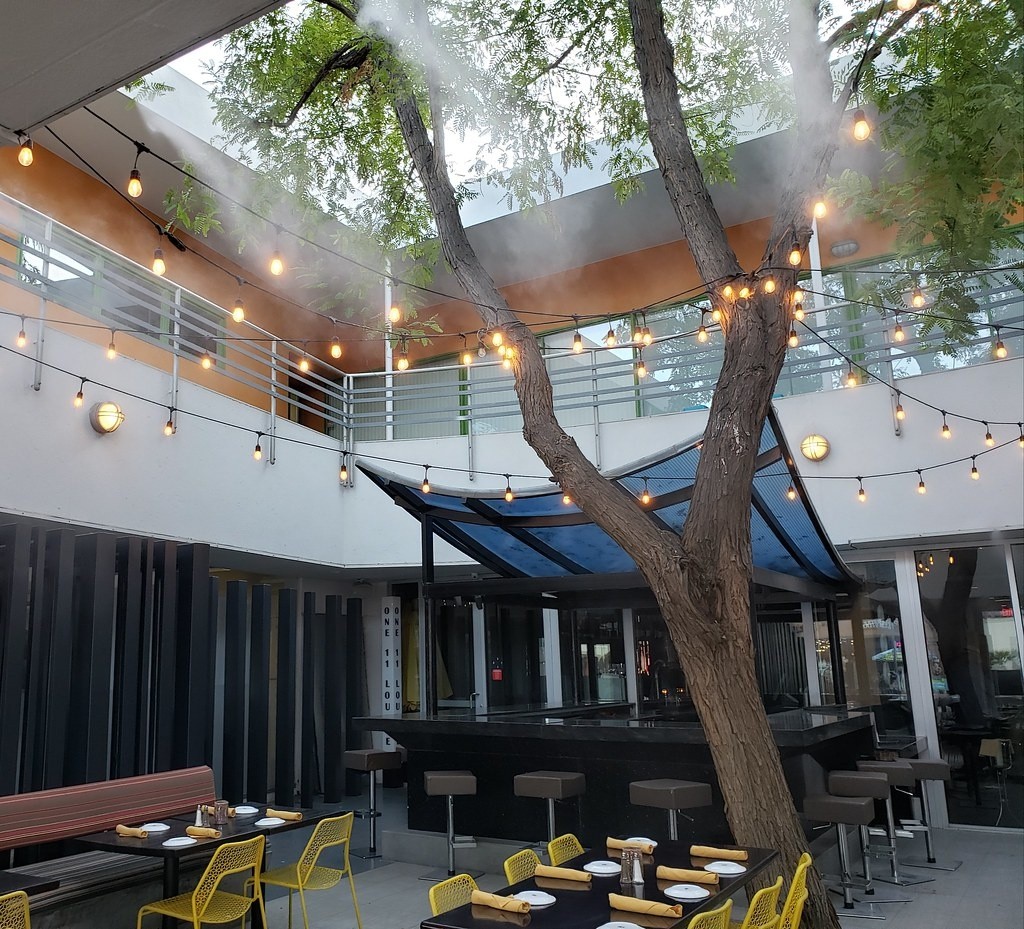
[74,802,345,927]
[0,870,61,901]
[420,834,777,929]
[351,700,877,844]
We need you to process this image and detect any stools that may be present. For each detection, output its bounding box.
[418,771,485,886]
[899,757,964,872]
[630,780,712,841]
[344,750,400,860]
[835,773,913,903]
[514,770,587,858]
[859,760,935,887]
[803,796,885,921]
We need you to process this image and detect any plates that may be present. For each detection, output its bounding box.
[139,823,171,831]
[513,891,556,905]
[596,921,645,929]
[162,837,198,846]
[583,861,621,873]
[529,903,556,910]
[718,874,743,878]
[665,894,710,903]
[625,837,658,846]
[235,806,259,813]
[703,861,747,874]
[254,818,285,826]
[664,884,710,898]
[590,873,620,878]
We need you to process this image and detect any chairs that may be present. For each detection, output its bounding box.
[0,891,32,929]
[136,834,267,928]
[504,850,545,885]
[547,832,587,867]
[243,812,364,929]
[686,853,813,929]
[429,874,480,917]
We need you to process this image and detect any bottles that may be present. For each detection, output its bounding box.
[631,851,645,884]
[194,805,203,826]
[620,883,632,896]
[633,884,644,900]
[619,851,632,884]
[203,805,210,827]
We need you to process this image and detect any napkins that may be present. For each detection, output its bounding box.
[116,825,147,837]
[608,893,682,918]
[536,864,593,882]
[186,826,223,839]
[605,836,651,855]
[691,846,747,860]
[471,889,530,913]
[265,809,302,821]
[200,805,236,819]
[657,865,721,883]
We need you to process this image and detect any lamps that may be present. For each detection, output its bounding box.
[801,431,827,460]
[88,401,123,435]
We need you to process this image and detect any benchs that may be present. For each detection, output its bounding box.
[0,766,273,913]
